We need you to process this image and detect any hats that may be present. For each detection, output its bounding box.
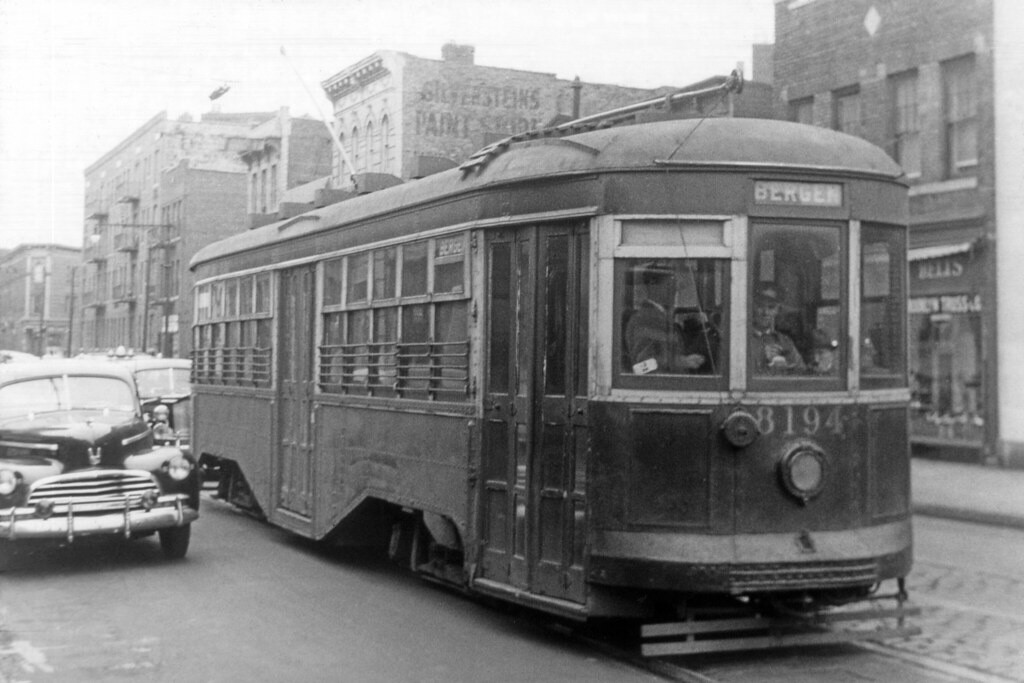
[803,329,838,350]
[755,281,785,303]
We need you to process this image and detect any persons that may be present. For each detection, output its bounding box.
[805,330,838,372]
[625,267,705,375]
[752,281,805,370]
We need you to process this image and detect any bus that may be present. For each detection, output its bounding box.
[193,118,914,655]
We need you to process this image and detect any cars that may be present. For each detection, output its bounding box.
[0,361,197,564]
[128,358,194,449]
